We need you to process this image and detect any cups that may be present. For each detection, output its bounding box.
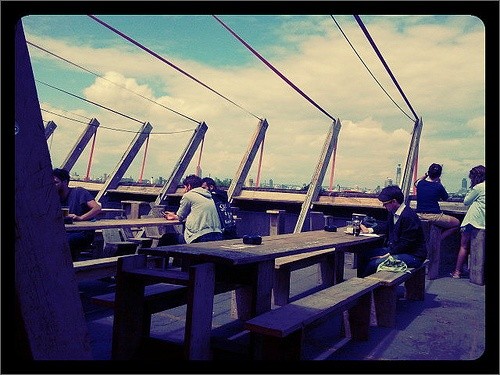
[61,208,69,217]
[346,221,353,233]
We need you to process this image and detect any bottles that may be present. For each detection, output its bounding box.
[352,216,360,236]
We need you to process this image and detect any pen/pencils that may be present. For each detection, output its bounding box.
[162,211,166,215]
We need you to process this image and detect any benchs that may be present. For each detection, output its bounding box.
[73,252,428,336]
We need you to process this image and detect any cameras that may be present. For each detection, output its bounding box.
[324,225,337,232]
[243,234,262,245]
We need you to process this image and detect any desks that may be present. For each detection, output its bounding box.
[65,218,189,239]
[138,227,387,336]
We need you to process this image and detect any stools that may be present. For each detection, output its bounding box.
[422,219,446,278]
[470,227,486,283]
[121,201,326,235]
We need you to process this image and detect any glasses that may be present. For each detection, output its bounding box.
[382,200,393,205]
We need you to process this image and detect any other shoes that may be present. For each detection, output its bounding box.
[450,271,461,279]
[423,257,432,265]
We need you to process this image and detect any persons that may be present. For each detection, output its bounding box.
[416,163,461,240]
[361,185,427,275]
[449,165,485,279]
[157,175,238,267]
[52,168,101,259]
